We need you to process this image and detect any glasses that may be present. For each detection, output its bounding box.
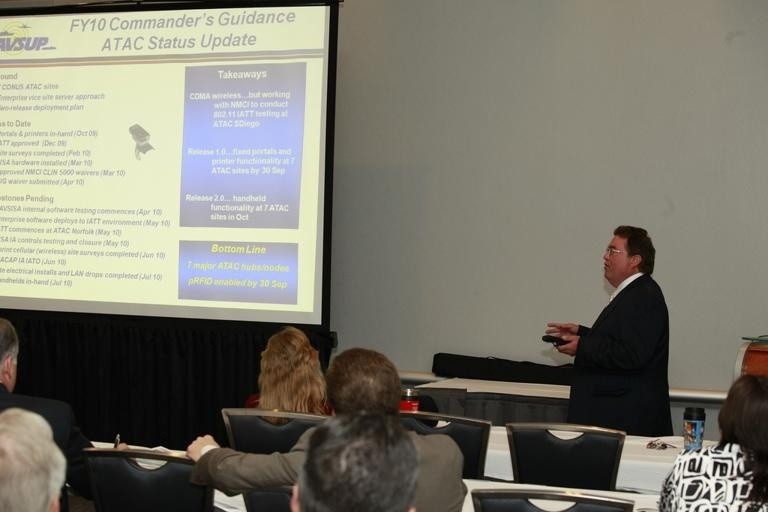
[606,247,625,256]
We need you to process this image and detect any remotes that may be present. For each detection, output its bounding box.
[542,335,570,345]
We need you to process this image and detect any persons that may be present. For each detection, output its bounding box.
[658,374,768,512]
[542,225,674,437]
[184,347,468,512]
[241,323,337,417]
[287,408,422,512]
[0,317,135,511]
[0,407,68,512]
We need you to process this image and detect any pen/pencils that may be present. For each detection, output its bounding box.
[113,435,119,449]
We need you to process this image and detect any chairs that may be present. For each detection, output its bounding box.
[222,408,329,511]
[81,447,214,511]
[398,411,634,511]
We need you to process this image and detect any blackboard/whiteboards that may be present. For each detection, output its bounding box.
[329,0,766,408]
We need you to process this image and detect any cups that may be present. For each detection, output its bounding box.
[398,389,420,418]
[683,406,707,452]
